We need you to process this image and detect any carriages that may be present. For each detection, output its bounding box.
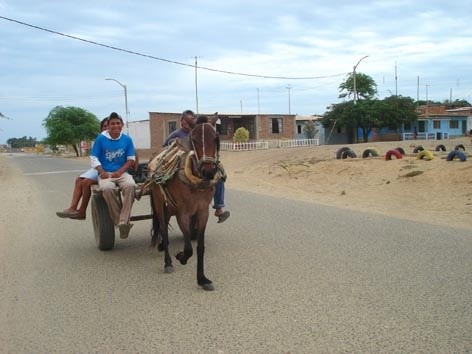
[91,112,227,291]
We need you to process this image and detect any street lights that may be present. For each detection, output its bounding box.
[353,55,369,142]
[104,78,128,136]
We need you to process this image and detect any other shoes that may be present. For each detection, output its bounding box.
[119,222,133,239]
[69,211,86,220]
[55,209,79,218]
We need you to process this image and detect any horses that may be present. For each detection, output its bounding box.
[149,112,220,292]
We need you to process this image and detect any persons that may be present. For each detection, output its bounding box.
[56,117,109,219]
[90,112,136,239]
[163,111,230,223]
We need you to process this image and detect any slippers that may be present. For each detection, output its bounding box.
[217,211,230,223]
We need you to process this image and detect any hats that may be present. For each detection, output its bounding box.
[107,113,123,125]
